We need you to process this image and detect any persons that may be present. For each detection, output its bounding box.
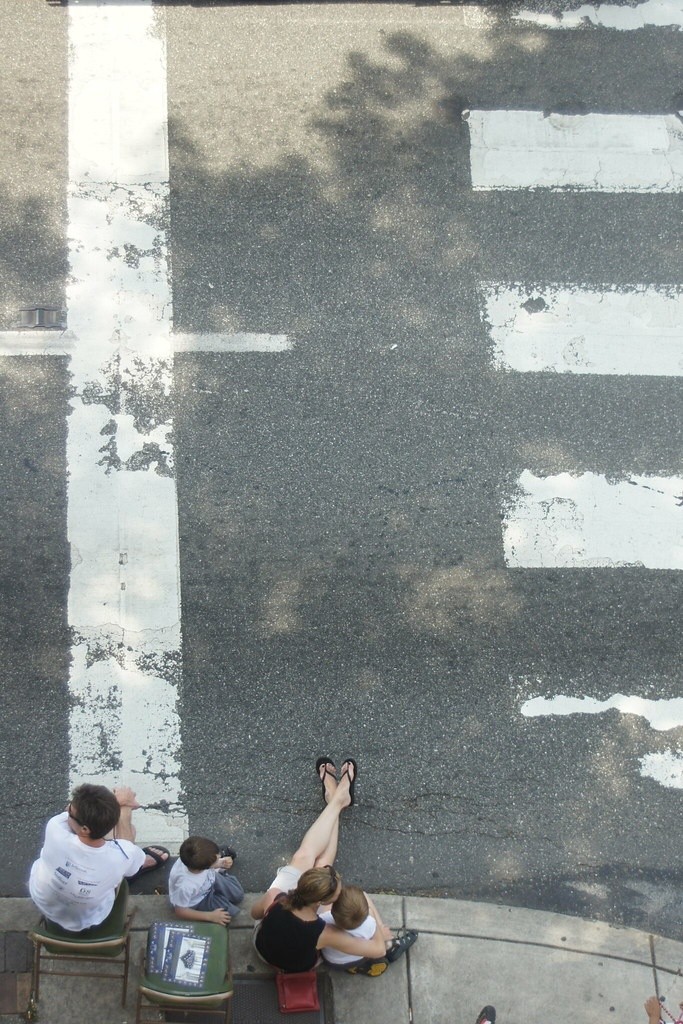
[477,1005,495,1024]
[169,837,243,925]
[28,784,169,931]
[250,758,386,968]
[318,888,417,968]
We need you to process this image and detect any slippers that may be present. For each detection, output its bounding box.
[127,845,170,881]
[339,758,357,807]
[315,756,337,801]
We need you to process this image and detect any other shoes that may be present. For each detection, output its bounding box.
[474,1005,496,1024]
[218,844,236,860]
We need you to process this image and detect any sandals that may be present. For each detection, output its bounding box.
[385,928,419,962]
[345,957,388,977]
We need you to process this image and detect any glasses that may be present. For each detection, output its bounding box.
[68,801,84,827]
[319,864,338,900]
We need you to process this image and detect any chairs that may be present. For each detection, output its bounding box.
[135,919,234,1023]
[20,880,137,1022]
